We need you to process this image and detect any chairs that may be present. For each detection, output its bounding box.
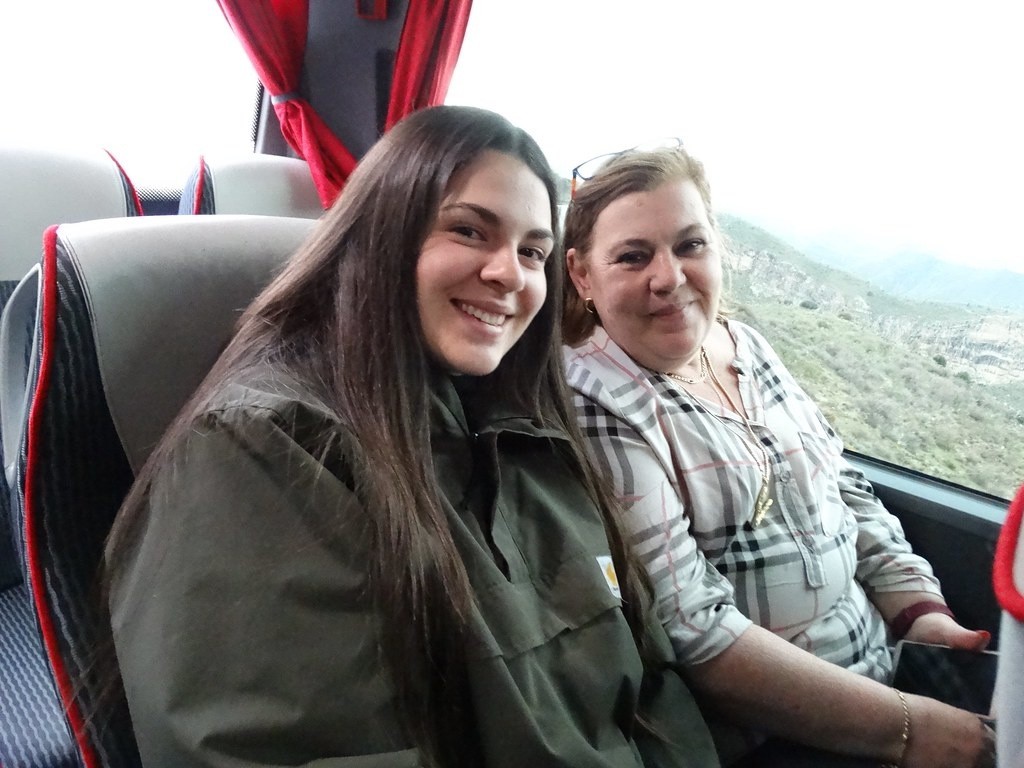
[176,154,333,223]
[0,145,140,768]
[0,214,338,768]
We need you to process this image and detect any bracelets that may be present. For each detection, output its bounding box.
[890,600,956,644]
[891,687,910,768]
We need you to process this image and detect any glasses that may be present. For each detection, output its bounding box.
[570,136,684,202]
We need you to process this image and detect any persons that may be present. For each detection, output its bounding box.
[101,105,721,768]
[560,146,997,768]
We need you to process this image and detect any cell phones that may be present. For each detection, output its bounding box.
[888,639,999,716]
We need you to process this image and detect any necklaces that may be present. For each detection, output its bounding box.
[661,345,774,530]
